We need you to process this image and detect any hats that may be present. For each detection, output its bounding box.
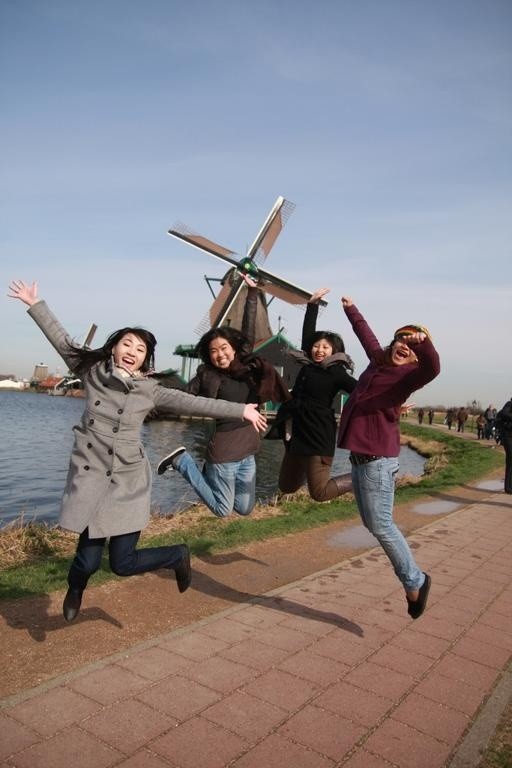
[394,323,431,341]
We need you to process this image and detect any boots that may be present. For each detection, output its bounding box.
[332,473,352,497]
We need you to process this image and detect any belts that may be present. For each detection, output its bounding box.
[348,454,380,464]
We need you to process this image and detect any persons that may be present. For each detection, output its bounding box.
[400,399,511,493]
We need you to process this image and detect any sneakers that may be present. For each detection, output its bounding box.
[158,446,206,476]
[64,595,81,622]
[174,544,192,593]
[407,572,432,619]
